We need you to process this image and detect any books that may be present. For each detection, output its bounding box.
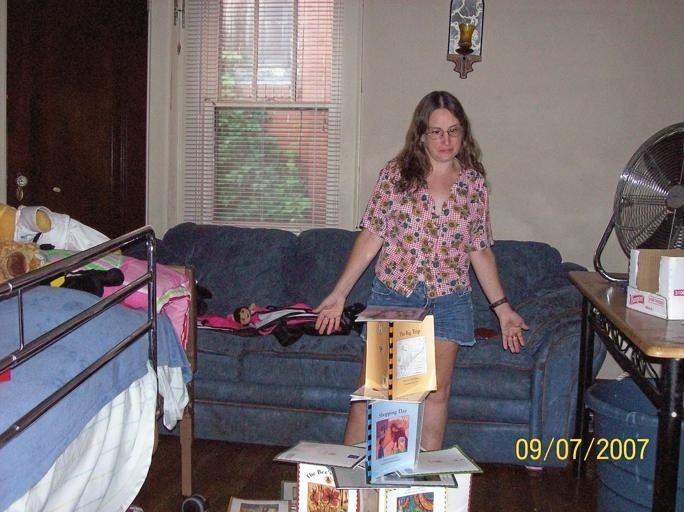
[365,400,425,484]
[352,305,431,323]
[364,313,438,397]
[349,385,433,404]
[228,439,489,511]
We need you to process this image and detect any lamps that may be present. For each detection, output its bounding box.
[456,22,475,57]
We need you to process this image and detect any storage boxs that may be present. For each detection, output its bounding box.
[626,248,684,320]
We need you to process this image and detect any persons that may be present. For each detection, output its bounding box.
[312,90,530,452]
[0,199,120,258]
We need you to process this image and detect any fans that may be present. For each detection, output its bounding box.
[594,123,684,286]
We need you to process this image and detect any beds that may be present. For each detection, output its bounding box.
[0,226,207,512]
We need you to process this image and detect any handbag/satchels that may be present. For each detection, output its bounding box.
[271,302,366,348]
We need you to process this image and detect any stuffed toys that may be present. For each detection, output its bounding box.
[43,266,125,297]
[0,239,39,284]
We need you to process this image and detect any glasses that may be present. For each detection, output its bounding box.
[424,126,464,141]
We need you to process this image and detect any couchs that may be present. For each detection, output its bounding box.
[120,223,609,472]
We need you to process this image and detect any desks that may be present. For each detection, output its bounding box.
[566,269,682,511]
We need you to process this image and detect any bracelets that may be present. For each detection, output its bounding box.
[488,296,509,309]
